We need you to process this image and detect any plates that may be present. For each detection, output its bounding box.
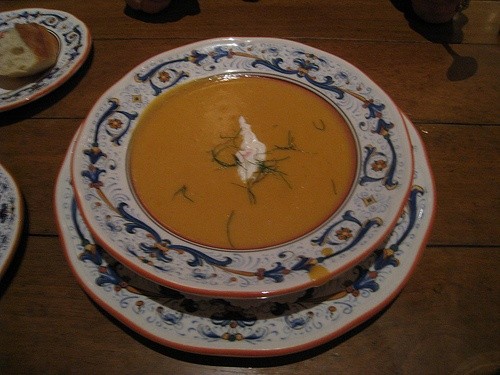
[54,108,437,358]
[72,35,415,300]
[1,165,21,274]
[0,8,91,112]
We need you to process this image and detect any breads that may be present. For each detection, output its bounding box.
[0,21,58,78]
